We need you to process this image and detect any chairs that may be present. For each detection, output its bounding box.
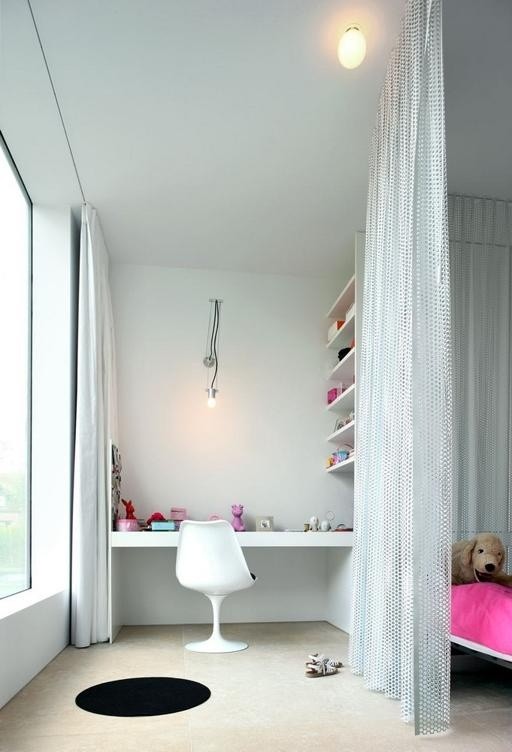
[176,519,255,655]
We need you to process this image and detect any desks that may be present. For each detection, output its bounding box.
[103,525,359,647]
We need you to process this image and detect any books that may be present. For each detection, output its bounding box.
[327,300,357,345]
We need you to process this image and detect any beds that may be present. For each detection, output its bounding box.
[447,574,512,670]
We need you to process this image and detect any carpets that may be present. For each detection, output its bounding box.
[75,677,211,717]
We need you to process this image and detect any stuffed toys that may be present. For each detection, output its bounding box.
[450,532,512,590]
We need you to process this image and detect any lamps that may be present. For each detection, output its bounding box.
[332,19,370,74]
[203,296,224,409]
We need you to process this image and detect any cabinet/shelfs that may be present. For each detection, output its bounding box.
[323,230,366,477]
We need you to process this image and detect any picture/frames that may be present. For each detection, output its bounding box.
[255,516,274,531]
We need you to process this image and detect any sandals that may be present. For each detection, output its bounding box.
[304,654,343,678]
